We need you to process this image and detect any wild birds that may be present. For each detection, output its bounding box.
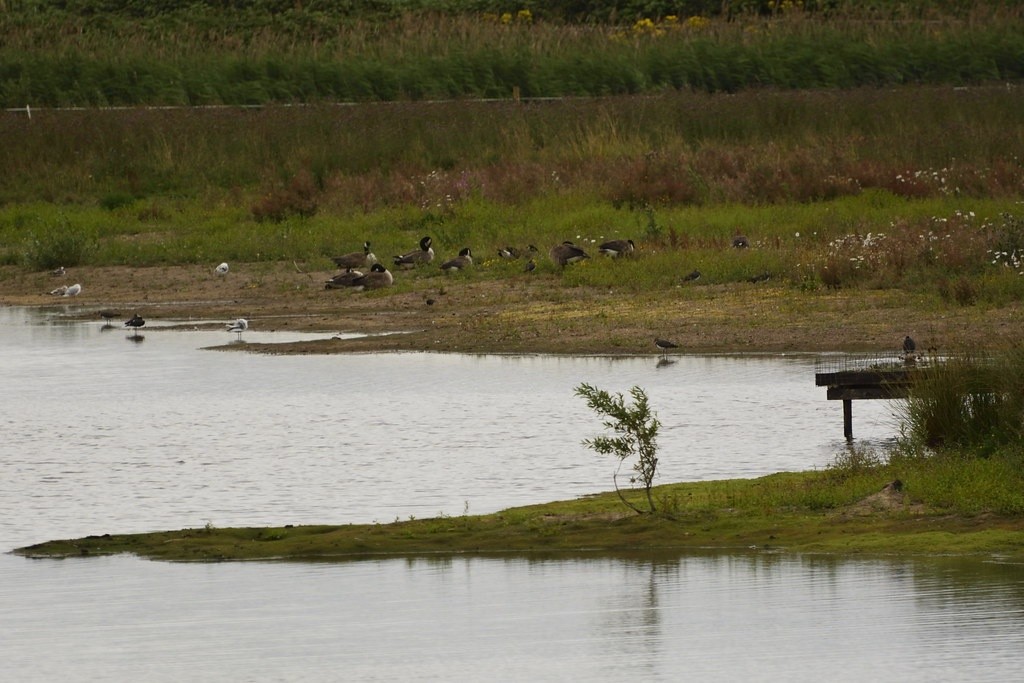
[731,236,749,251]
[213,262,229,275]
[902,335,915,354]
[652,338,679,352]
[124,313,145,328]
[737,273,769,285]
[324,235,638,292]
[46,283,81,299]
[225,318,248,336]
[679,268,702,286]
[97,310,121,322]
